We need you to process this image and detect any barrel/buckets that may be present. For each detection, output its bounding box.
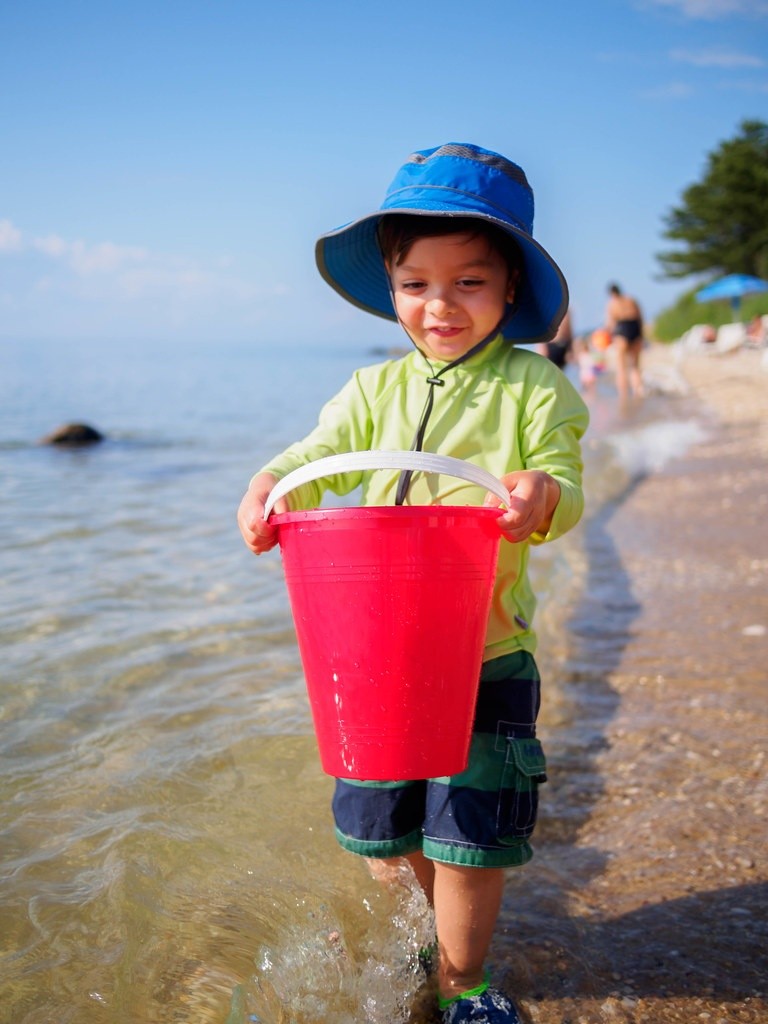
[263,450,511,778]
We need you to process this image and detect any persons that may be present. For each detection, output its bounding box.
[535,284,763,411]
[235,145,590,1024]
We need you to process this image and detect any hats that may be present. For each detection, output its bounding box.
[315,142,569,345]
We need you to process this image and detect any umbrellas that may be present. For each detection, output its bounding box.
[694,273,768,325]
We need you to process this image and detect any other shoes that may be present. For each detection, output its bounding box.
[418,943,439,984]
[434,960,523,1024]
